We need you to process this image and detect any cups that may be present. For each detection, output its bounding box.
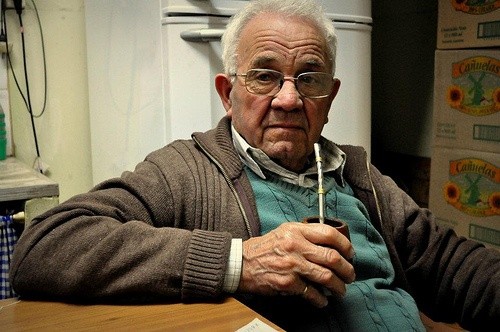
[301,214,354,296]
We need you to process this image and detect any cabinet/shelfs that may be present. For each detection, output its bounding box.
[0,155,60,298]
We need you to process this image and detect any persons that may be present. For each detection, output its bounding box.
[10,0,500,332]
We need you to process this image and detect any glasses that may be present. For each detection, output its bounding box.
[228,69,336,98]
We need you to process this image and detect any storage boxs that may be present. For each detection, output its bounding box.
[433,49,500,153]
[436,0,500,49]
[427,147,500,254]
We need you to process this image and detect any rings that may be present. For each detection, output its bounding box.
[301,287,307,294]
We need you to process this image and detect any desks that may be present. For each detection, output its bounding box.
[0,295,285,332]
